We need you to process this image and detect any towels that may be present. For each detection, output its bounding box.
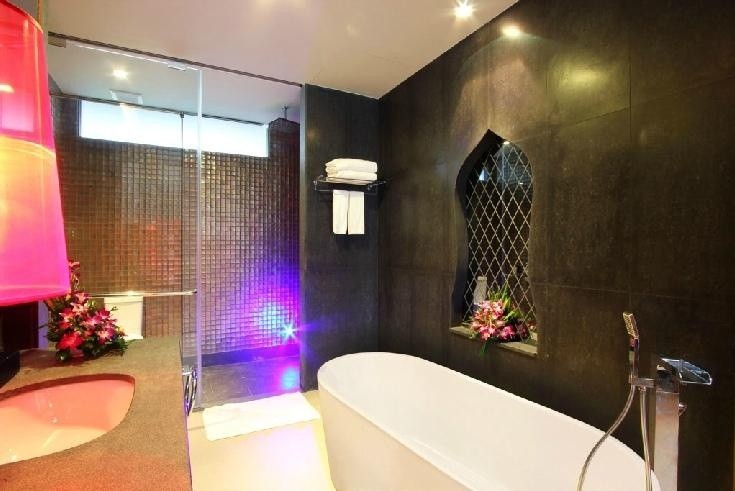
[325,156,378,235]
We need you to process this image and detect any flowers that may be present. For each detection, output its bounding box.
[38,262,126,361]
[460,284,536,359]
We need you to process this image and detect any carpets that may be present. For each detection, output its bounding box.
[201,392,321,442]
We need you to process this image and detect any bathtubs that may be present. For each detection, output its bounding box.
[316,351,661,491]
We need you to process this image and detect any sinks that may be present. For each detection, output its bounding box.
[0,372,135,465]
